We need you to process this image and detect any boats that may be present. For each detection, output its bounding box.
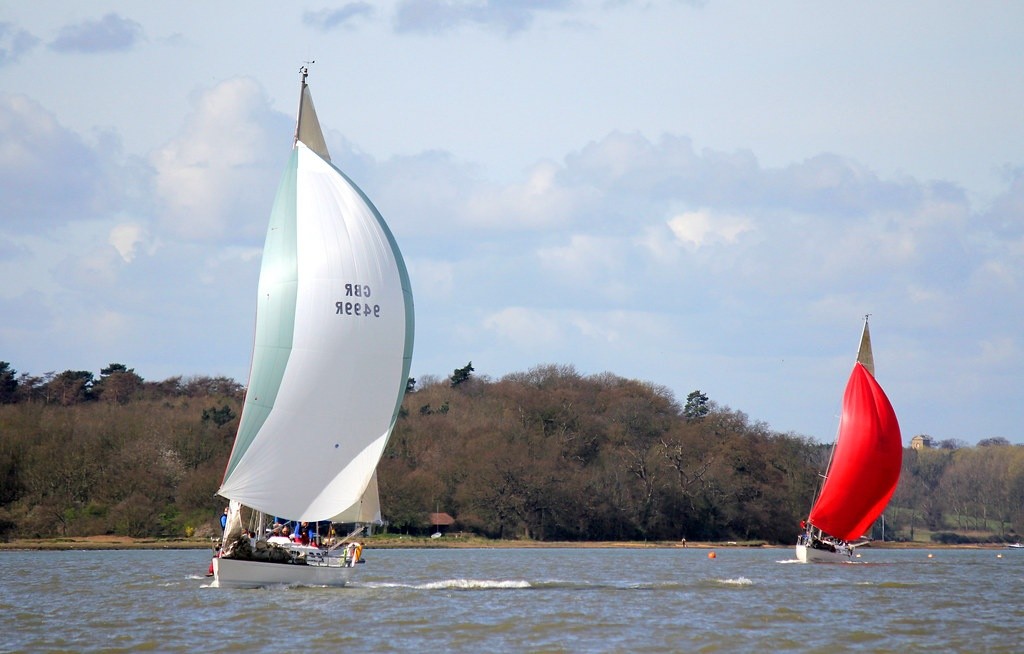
[1008,543,1024,549]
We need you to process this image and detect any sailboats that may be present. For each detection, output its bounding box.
[796,313,903,564]
[205,68,415,588]
[431,507,442,539]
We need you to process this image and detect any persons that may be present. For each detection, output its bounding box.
[220,507,229,534]
[269,521,313,547]
[242,528,256,547]
[325,533,336,548]
[800,519,852,555]
[682,537,686,548]
